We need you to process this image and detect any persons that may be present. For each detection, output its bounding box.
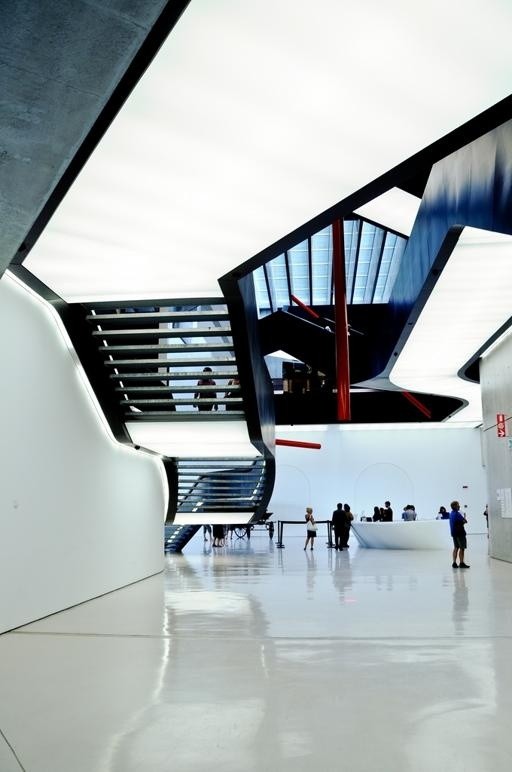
[449,499,470,568]
[192,367,218,411]
[436,506,450,519]
[332,503,347,551]
[223,379,243,410]
[202,525,211,541]
[212,524,224,547]
[303,506,316,550]
[372,501,416,522]
[343,504,354,548]
[483,504,488,538]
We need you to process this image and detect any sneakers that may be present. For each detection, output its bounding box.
[453,563,469,568]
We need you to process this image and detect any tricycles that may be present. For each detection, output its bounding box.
[234,509,275,539]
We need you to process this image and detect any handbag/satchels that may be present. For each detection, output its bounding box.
[306,521,317,531]
